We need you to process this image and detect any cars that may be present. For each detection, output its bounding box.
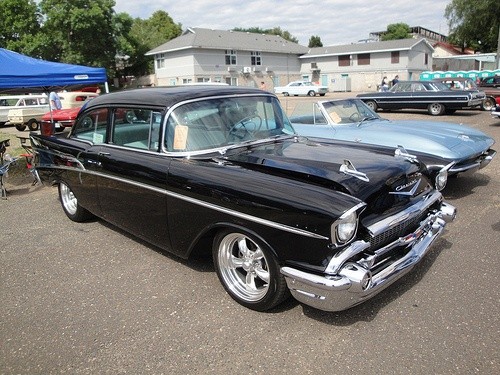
[0,91,127,132]
[29,84,458,314]
[477,75,500,111]
[274,80,329,97]
[431,77,482,110]
[355,79,486,117]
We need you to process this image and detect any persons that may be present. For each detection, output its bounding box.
[40,92,47,103]
[392,75,399,86]
[475,77,480,85]
[259,81,264,89]
[381,76,388,92]
[450,80,456,88]
[493,74,497,83]
[96,86,101,93]
[49,92,62,111]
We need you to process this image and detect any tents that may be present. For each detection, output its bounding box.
[0,48,110,134]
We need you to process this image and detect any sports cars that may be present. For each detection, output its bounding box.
[234,96,497,179]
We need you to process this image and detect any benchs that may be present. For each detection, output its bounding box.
[76,123,160,150]
